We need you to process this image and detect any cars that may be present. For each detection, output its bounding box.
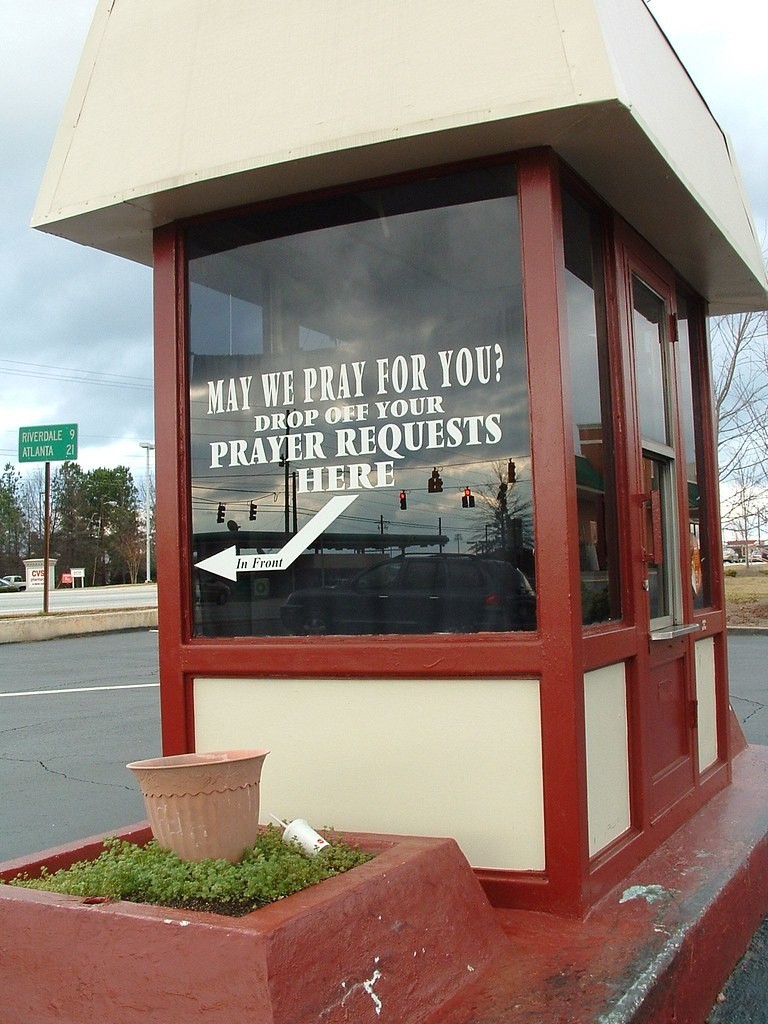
[0,578,19,593]
[751,551,763,562]
[722,547,739,564]
[197,570,231,606]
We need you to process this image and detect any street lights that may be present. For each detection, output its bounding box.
[138,442,155,583]
[39,491,45,558]
[101,501,117,585]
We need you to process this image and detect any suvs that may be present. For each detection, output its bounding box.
[279,552,537,635]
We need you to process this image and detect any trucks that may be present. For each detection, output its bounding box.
[3,576,26,591]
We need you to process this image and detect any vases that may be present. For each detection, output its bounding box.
[125,748,271,868]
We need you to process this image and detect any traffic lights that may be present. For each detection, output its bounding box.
[469,496,475,507]
[216,505,225,523]
[399,492,407,510]
[465,489,470,496]
[462,496,468,508]
[249,503,258,521]
